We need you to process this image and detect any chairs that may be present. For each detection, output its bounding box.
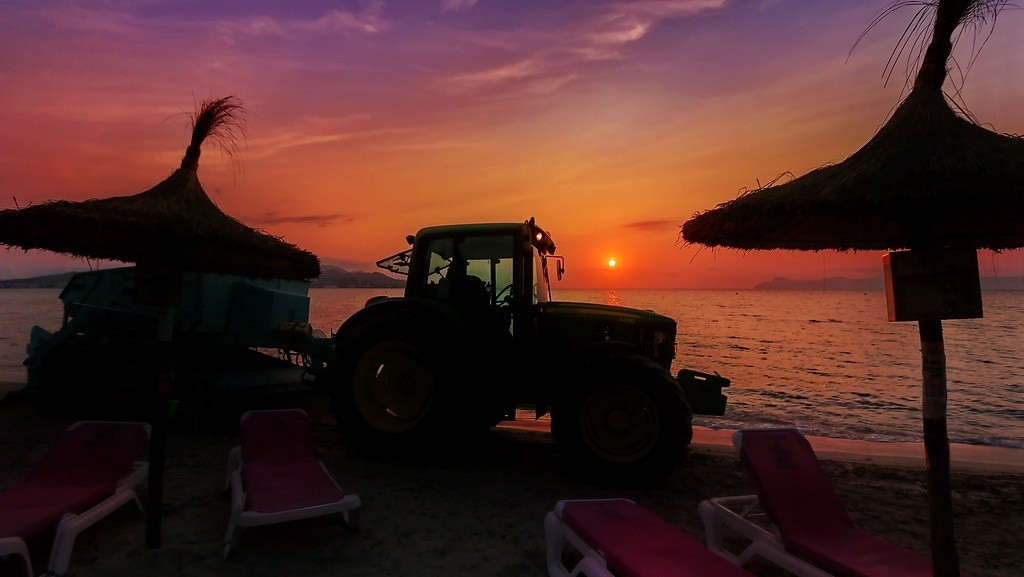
[221,409,363,560]
[543,498,755,577]
[0,422,151,577]
[696,429,972,577]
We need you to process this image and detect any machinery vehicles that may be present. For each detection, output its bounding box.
[269,217,733,493]
